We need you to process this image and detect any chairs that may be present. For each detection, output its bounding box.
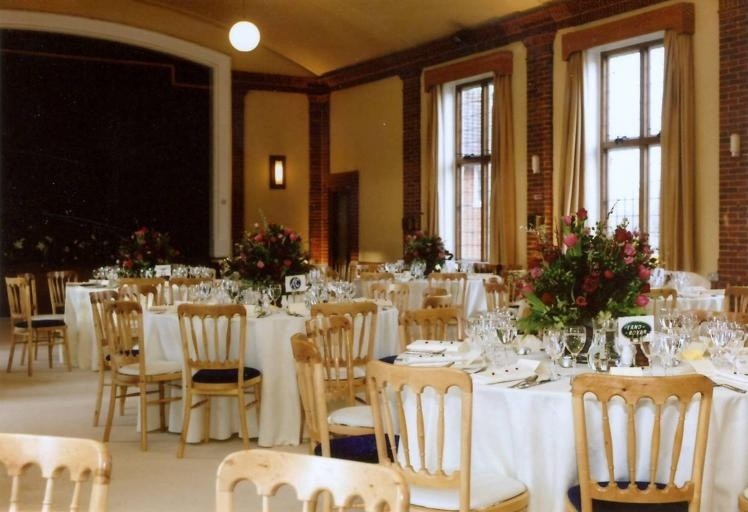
[377,272,396,280]
[15,272,65,366]
[366,365,530,512]
[117,276,165,307]
[176,302,265,460]
[722,284,748,313]
[482,279,507,312]
[337,257,358,283]
[305,314,390,436]
[100,297,185,452]
[296,300,380,445]
[359,271,381,299]
[168,276,215,305]
[645,287,678,319]
[47,269,78,353]
[89,290,142,427]
[564,372,715,511]
[0,431,113,512]
[420,286,447,297]
[421,293,455,344]
[367,261,378,273]
[214,447,394,512]
[5,276,72,378]
[427,271,469,342]
[394,304,467,356]
[288,331,376,461]
[368,281,412,350]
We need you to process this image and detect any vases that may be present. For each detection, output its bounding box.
[558,316,594,364]
[424,264,435,275]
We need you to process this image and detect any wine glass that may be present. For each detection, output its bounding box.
[376,263,397,274]
[466,308,515,375]
[304,268,355,304]
[258,288,274,313]
[700,316,745,375]
[268,283,282,309]
[542,328,565,381]
[642,308,694,373]
[170,266,212,280]
[562,325,586,374]
[188,284,211,304]
[217,279,238,304]
[91,266,118,289]
[412,261,427,281]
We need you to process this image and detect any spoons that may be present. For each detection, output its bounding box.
[507,375,538,388]
[711,381,747,394]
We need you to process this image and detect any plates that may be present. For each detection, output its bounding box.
[405,360,454,369]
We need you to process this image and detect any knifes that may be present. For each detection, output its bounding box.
[518,379,551,389]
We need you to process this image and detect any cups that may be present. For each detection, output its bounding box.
[588,320,608,370]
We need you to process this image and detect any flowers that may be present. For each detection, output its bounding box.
[508,199,668,342]
[402,231,449,271]
[113,226,180,278]
[222,222,317,286]
